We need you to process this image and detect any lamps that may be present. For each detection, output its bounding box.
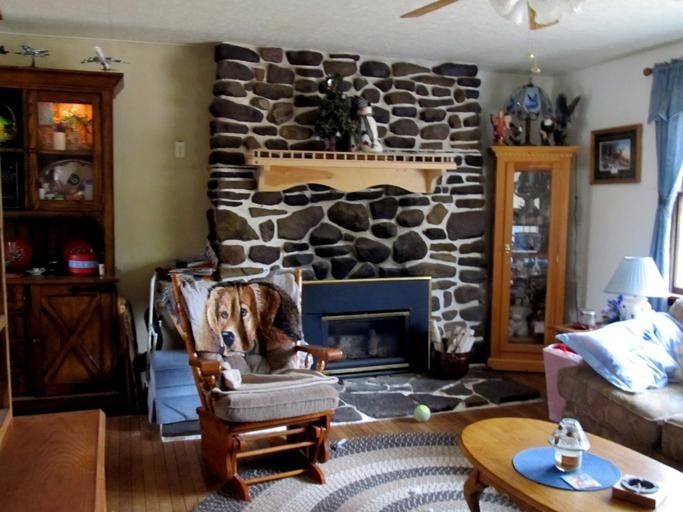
[601,255,671,322]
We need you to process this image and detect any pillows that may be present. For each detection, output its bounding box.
[554,314,683,392]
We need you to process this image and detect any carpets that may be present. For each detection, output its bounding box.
[160,366,543,444]
[191,433,522,512]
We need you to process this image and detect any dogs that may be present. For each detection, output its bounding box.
[191,280,300,374]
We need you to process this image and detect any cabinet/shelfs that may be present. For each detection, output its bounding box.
[485,145,582,374]
[0,67,133,417]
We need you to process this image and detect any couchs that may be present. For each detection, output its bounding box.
[556,295,683,471]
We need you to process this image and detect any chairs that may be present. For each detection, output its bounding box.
[169,263,345,503]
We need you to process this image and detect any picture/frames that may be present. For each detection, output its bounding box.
[589,123,643,184]
